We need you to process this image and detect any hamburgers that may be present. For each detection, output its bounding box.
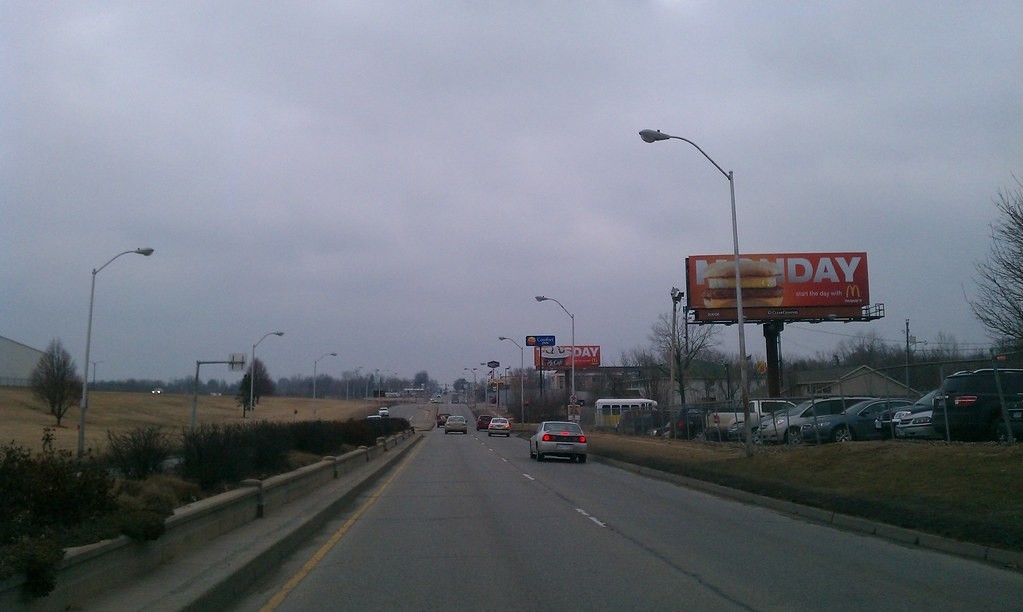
[701,261,784,308]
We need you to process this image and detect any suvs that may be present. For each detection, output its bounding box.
[932,368,1023,440]
[758,397,871,446]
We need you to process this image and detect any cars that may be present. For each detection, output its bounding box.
[488,418,512,435]
[528,421,588,463]
[378,407,389,415]
[998,394,1023,441]
[476,415,494,431]
[896,411,934,440]
[874,389,938,439]
[437,413,452,428]
[444,415,468,434]
[368,416,381,420]
[800,397,915,445]
[617,406,738,442]
[430,389,459,406]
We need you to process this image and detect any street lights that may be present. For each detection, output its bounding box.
[535,294,576,425]
[504,366,510,407]
[88,360,104,389]
[498,336,525,424]
[363,368,400,403]
[313,353,340,415]
[75,246,157,466]
[668,286,685,439]
[464,367,477,408]
[480,362,489,412]
[636,127,754,455]
[249,330,284,421]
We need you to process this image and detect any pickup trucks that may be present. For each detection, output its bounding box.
[704,397,799,442]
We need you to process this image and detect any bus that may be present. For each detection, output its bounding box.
[594,399,658,431]
